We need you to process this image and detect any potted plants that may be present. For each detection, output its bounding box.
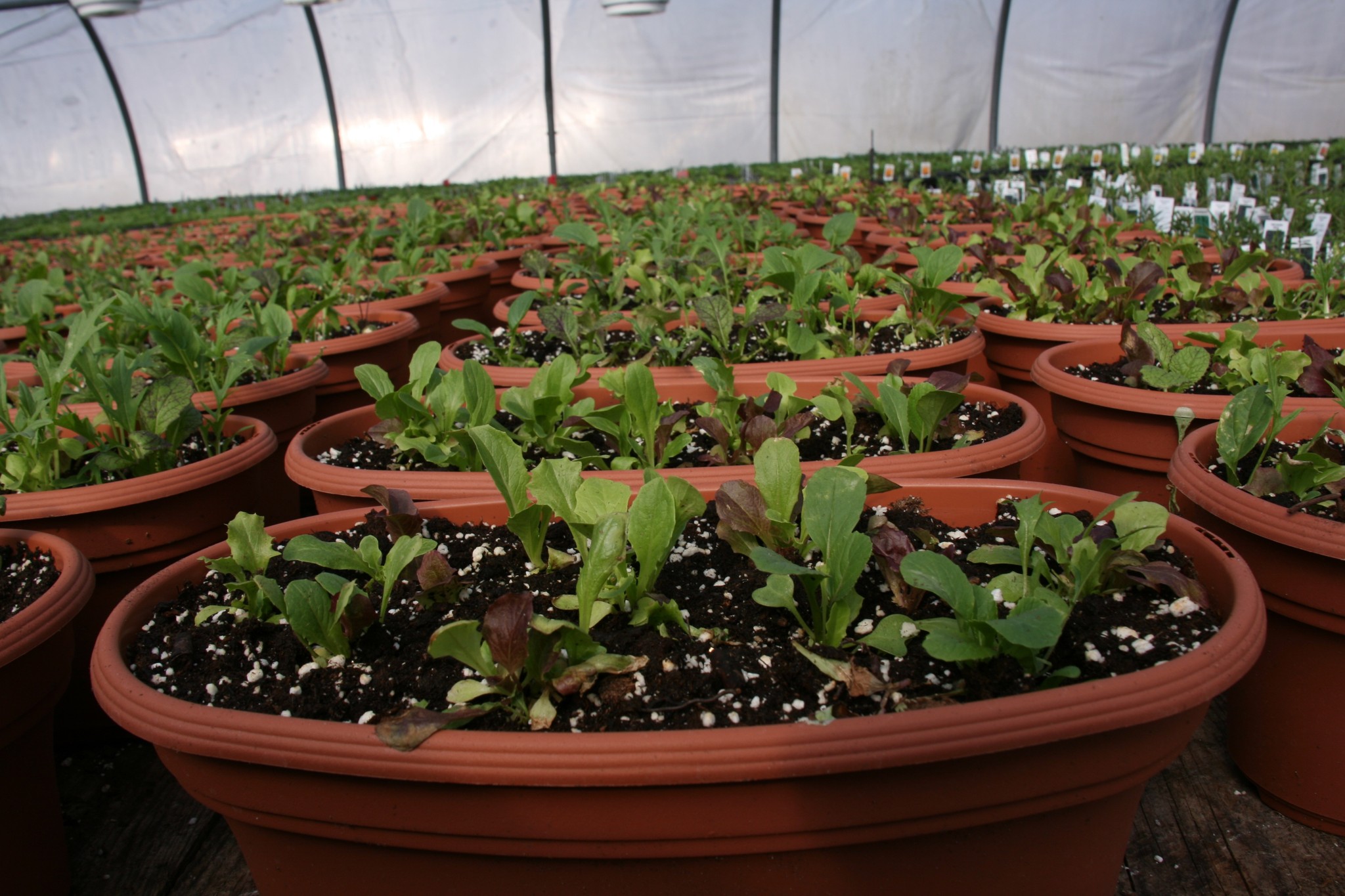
[0,182,1345,896]
